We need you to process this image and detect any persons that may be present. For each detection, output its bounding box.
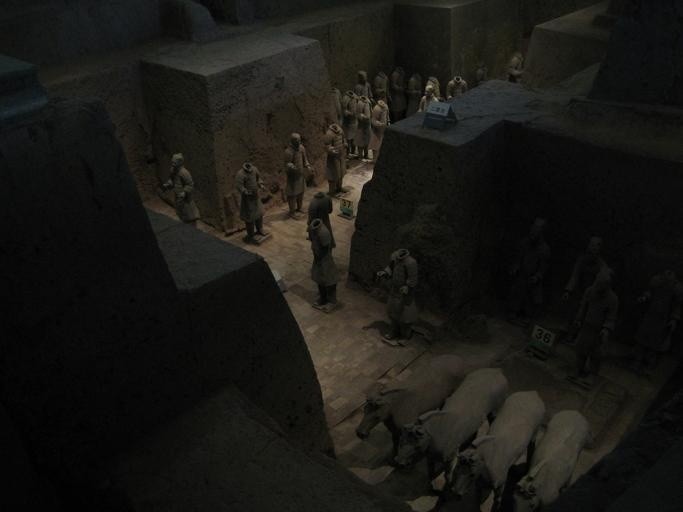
[238,163,269,240]
[377,249,417,338]
[309,218,341,307]
[163,153,200,227]
[510,212,681,383]
[335,67,441,162]
[447,76,467,100]
[285,133,311,216]
[325,123,350,195]
[307,192,336,248]
